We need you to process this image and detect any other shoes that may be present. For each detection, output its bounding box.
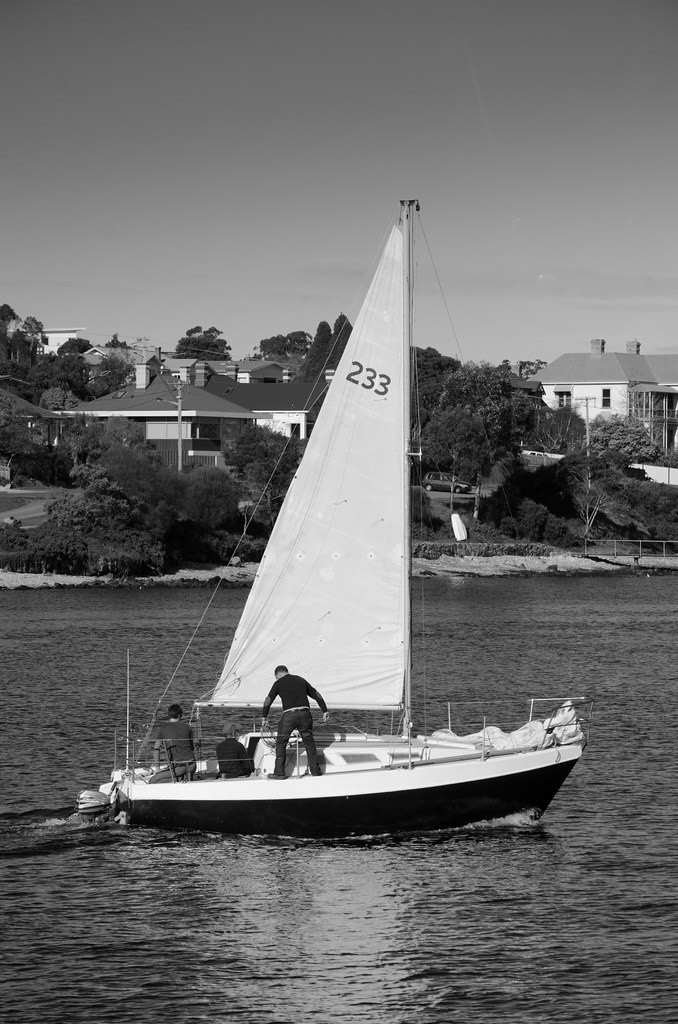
[267,774,285,780]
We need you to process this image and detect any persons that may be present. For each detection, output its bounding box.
[148,704,197,783]
[216,724,254,779]
[262,665,329,780]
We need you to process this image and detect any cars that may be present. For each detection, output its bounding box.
[422,470,473,494]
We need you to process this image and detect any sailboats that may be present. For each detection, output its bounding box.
[76,200,588,836]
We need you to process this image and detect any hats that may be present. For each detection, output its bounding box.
[222,723,237,733]
